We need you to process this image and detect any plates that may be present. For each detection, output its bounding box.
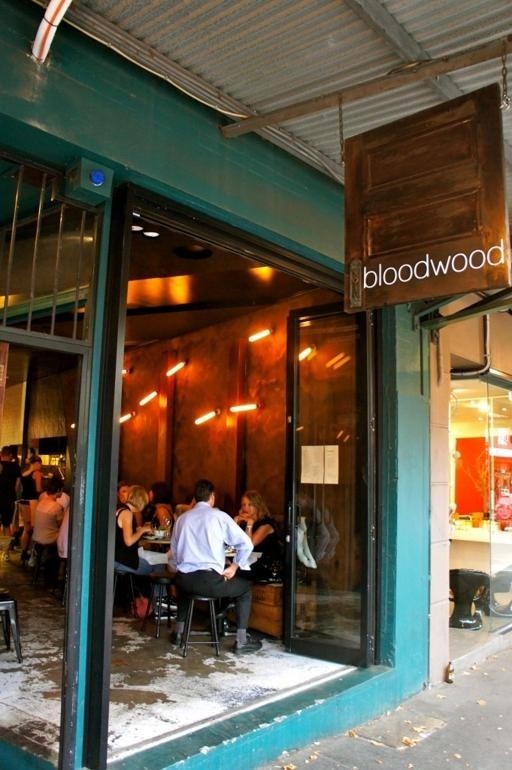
[143,535,159,539]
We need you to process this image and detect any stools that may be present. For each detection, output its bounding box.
[450,569,489,631]
[484,564,512,617]
[0,549,223,663]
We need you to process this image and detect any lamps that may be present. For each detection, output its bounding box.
[119,327,316,425]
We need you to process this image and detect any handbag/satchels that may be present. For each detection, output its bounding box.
[251,552,306,587]
[130,592,153,618]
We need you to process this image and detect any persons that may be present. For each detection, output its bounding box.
[0,446,71,589]
[115,479,284,654]
[297,491,341,640]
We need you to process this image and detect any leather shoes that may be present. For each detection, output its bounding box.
[215,600,237,632]
[169,630,182,645]
[233,638,262,654]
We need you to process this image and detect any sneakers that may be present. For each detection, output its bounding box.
[155,597,179,620]
[2,551,35,567]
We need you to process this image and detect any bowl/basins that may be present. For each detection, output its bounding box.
[154,530,166,539]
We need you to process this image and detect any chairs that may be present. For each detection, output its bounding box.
[225,520,317,634]
